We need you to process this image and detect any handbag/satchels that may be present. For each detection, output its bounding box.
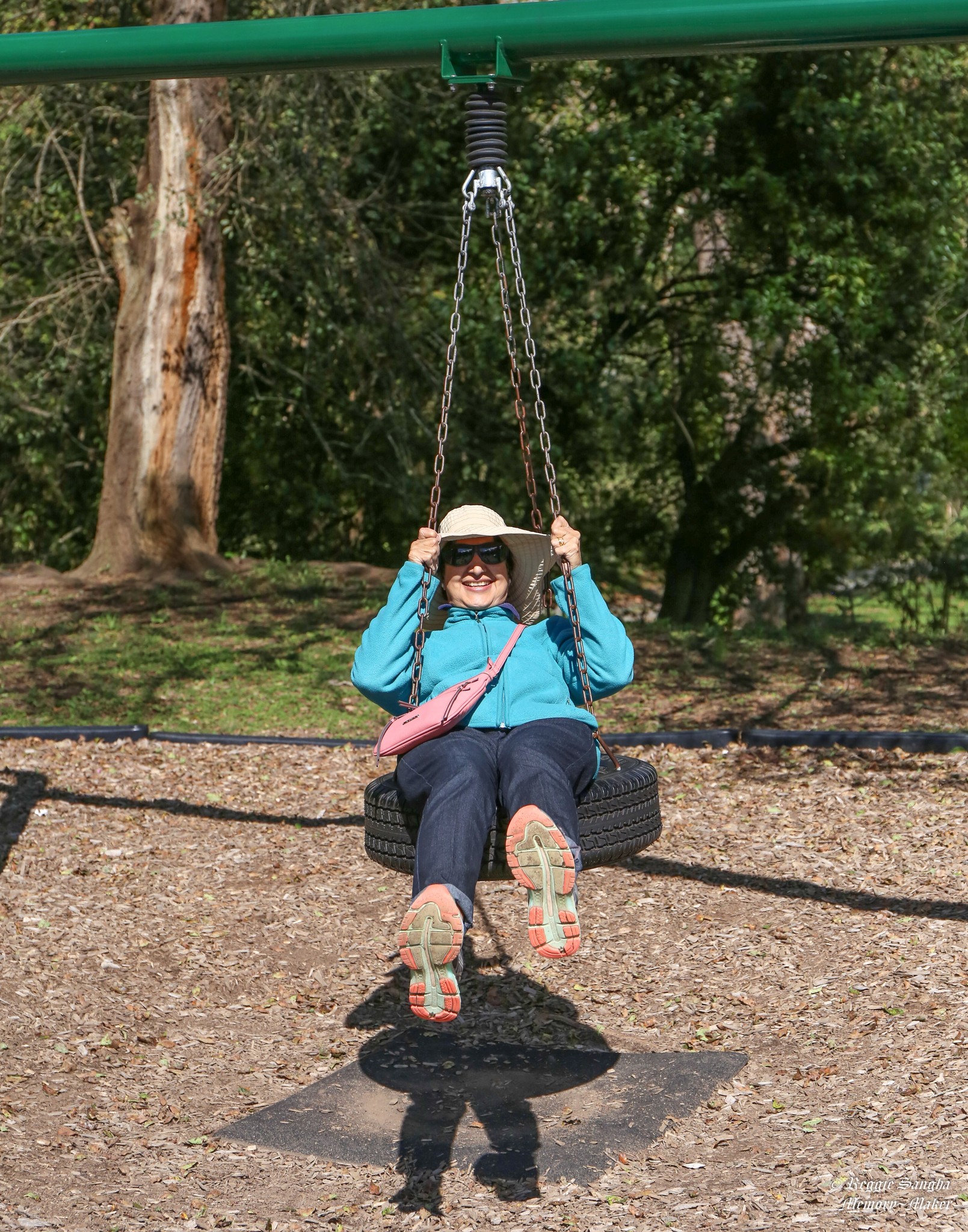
[372,672,492,767]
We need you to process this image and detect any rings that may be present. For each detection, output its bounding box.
[558,538,562,546]
[561,537,564,545]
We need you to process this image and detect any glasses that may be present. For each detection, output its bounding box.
[440,540,510,566]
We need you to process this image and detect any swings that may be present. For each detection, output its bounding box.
[360,76,665,885]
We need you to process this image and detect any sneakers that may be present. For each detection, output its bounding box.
[398,884,464,1022]
[505,805,581,959]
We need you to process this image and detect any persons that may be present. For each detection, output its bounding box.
[350,505,635,1022]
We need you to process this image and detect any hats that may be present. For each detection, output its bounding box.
[419,505,561,630]
